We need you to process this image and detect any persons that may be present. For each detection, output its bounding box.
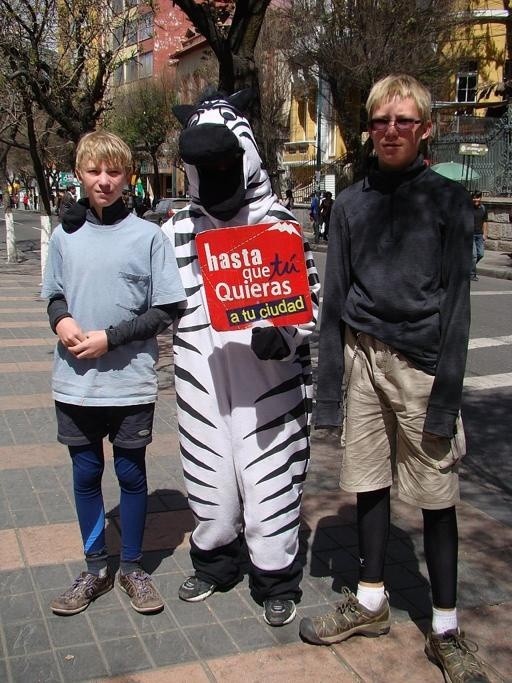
[470,189,488,281]
[120,187,185,218]
[308,188,334,242]
[41,131,187,615]
[300,75,489,682]
[9,184,77,224]
[286,186,293,211]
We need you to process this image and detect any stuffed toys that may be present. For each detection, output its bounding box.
[157,98,323,626]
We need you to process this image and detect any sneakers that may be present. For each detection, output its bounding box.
[116,568,165,614]
[51,564,116,614]
[262,599,297,627]
[298,589,392,646]
[178,573,216,603]
[422,626,492,682]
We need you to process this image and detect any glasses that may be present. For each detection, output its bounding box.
[366,114,423,132]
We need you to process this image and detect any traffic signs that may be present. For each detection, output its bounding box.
[459,143,489,156]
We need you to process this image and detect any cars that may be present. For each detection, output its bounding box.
[141,196,192,226]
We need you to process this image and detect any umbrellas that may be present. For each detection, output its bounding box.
[431,160,480,182]
